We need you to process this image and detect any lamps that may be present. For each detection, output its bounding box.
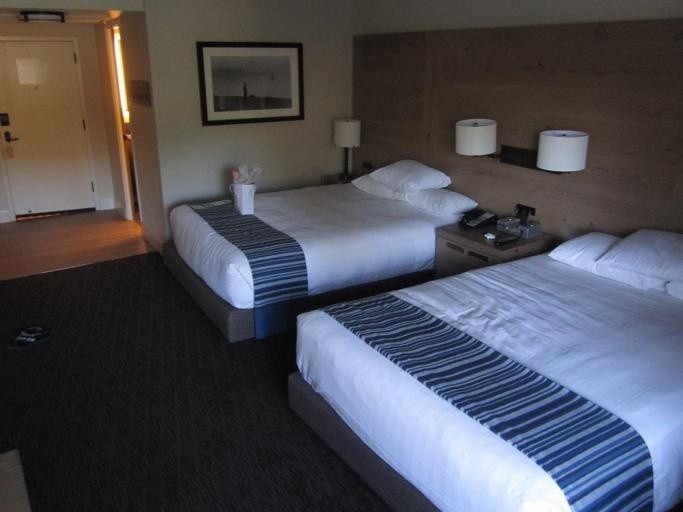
[332,116,360,178]
[452,117,588,175]
[14,10,65,25]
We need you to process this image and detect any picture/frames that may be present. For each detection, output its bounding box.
[195,40,306,126]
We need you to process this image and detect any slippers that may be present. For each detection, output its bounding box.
[6,326,52,350]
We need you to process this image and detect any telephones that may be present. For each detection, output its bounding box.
[461,207,497,229]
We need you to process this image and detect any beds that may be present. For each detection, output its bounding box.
[162,180,467,344]
[287,252,682,512]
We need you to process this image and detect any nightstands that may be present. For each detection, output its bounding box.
[321,172,359,186]
[432,218,556,278]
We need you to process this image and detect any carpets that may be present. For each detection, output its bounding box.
[0,254,388,512]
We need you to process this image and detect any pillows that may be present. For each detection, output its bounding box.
[546,230,667,293]
[368,158,452,194]
[352,173,409,202]
[664,281,683,301]
[590,226,682,283]
[393,188,478,214]
